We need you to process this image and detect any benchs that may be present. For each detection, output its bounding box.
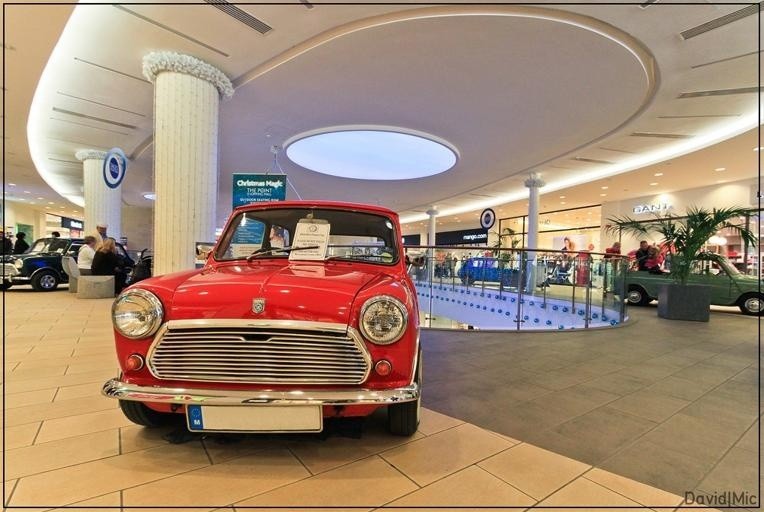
[61,254,115,298]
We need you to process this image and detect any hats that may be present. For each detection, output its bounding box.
[97,221,109,229]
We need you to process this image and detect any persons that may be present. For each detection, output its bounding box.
[560,237,574,255]
[635,241,660,271]
[270,225,286,257]
[90,226,108,253]
[603,242,621,259]
[44,232,60,253]
[91,237,127,298]
[77,235,96,276]
[442,252,473,277]
[13,231,30,254]
[0,231,13,255]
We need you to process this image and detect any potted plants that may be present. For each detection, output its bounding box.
[604,206,758,322]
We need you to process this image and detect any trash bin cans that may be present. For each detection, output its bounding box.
[416,265,427,282]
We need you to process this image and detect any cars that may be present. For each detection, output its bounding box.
[100,199,422,440]
[457,258,520,288]
[1,236,137,291]
[611,253,764,316]
